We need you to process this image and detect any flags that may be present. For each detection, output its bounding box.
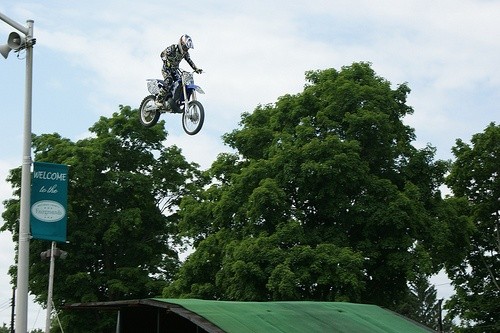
[31,161,69,244]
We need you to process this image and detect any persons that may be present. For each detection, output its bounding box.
[155,34,203,102]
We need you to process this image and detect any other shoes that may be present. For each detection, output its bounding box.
[164,92,171,101]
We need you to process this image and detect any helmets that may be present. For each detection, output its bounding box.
[177,34,194,54]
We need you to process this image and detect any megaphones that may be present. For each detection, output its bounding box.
[7,32,27,50]
[0,42,12,59]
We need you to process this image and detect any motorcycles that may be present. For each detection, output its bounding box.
[139,65,205,135]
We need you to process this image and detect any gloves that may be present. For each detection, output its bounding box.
[194,67,203,74]
[169,63,176,73]
